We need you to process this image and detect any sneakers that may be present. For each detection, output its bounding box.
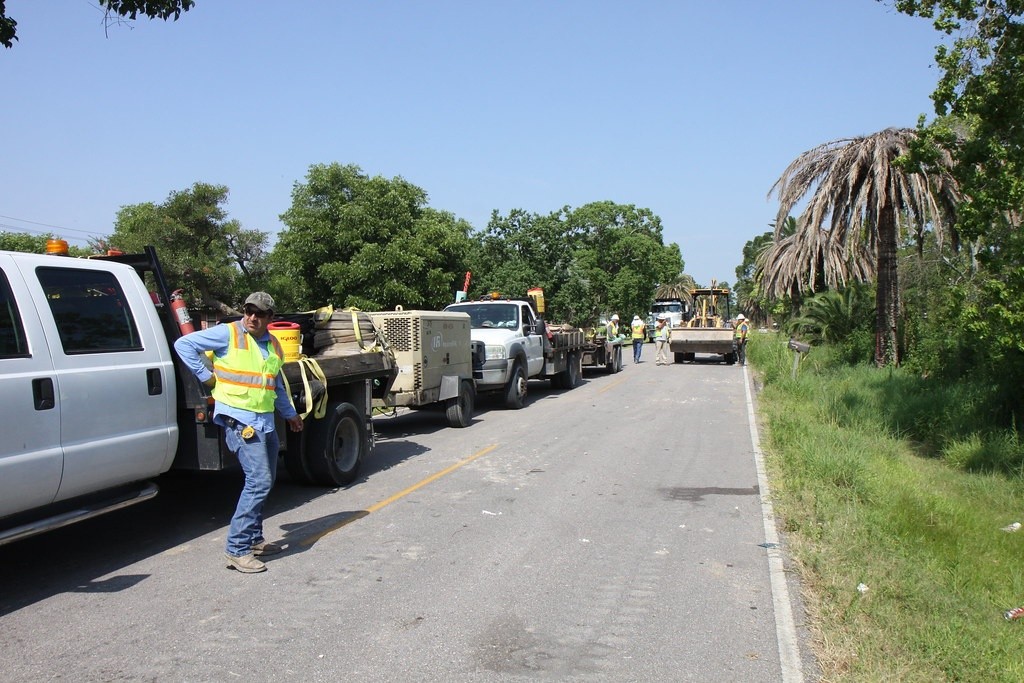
[250,541,283,555]
[224,552,267,572]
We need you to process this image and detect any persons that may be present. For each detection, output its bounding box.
[607,314,619,341]
[726,313,751,366]
[631,316,646,364]
[655,316,670,366]
[174,292,304,573]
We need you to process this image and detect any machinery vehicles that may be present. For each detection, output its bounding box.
[668,276,739,365]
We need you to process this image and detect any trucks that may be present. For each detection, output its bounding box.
[0,239,392,548]
[648,297,689,344]
[443,291,598,409]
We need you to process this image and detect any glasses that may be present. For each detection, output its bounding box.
[244,307,270,317]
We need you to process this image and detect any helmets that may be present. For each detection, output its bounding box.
[657,313,666,319]
[634,316,639,319]
[611,314,619,320]
[745,318,749,322]
[736,314,745,319]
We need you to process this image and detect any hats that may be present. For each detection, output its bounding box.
[243,292,276,317]
[482,320,493,326]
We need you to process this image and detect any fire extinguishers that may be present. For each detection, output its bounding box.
[170,288,195,335]
[545,321,552,339]
